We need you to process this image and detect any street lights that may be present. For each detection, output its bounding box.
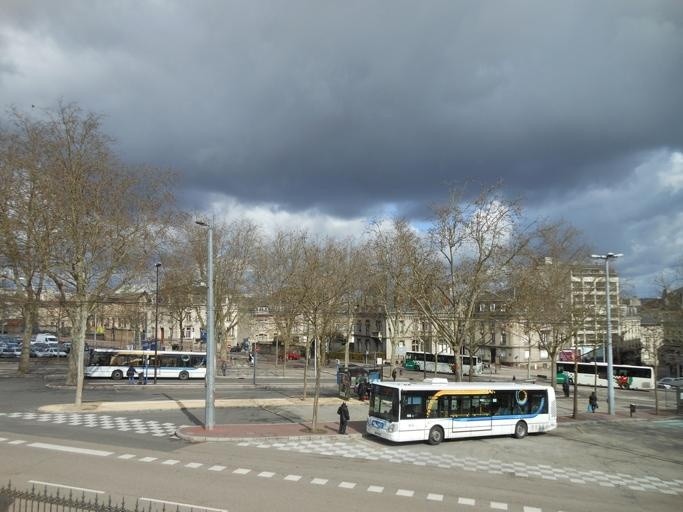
[154,262,163,384]
[195,222,215,430]
[587,252,624,416]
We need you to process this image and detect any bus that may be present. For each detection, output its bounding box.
[402,351,483,377]
[84,348,206,379]
[556,361,656,391]
[367,377,558,446]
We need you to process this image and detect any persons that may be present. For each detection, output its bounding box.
[392,367,396,381]
[562,376,569,397]
[588,391,597,413]
[220,360,226,377]
[336,401,349,434]
[126,364,137,385]
[354,378,372,401]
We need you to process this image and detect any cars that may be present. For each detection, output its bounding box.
[279,352,299,360]
[0,332,89,358]
[657,377,683,388]
